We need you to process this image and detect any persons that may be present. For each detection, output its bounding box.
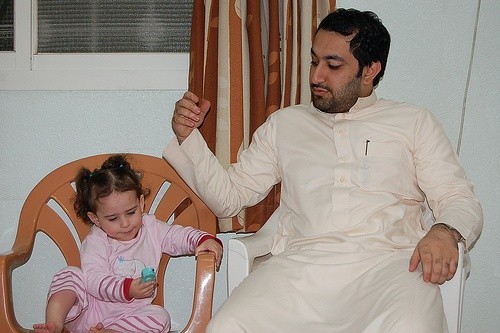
[161,9,484,333]
[33,154,224,333]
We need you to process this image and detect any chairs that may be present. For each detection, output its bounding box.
[0,153,216,333]
[227,205,465,333]
